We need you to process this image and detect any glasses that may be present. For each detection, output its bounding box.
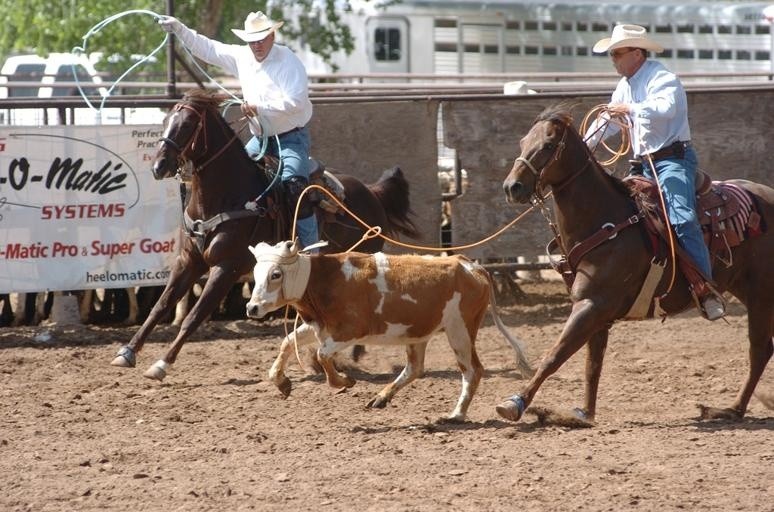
[610,48,635,58]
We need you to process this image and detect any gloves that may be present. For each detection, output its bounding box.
[154,15,182,34]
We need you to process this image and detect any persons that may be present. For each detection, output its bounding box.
[578,24,725,322]
[156,9,321,255]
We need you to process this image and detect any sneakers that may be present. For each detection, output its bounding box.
[700,292,724,320]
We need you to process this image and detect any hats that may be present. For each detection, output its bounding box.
[592,24,665,53]
[231,11,284,42]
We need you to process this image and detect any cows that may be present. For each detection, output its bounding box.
[245,235,535,425]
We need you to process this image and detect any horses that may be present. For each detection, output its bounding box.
[109,88,424,383]
[495,101,774,428]
